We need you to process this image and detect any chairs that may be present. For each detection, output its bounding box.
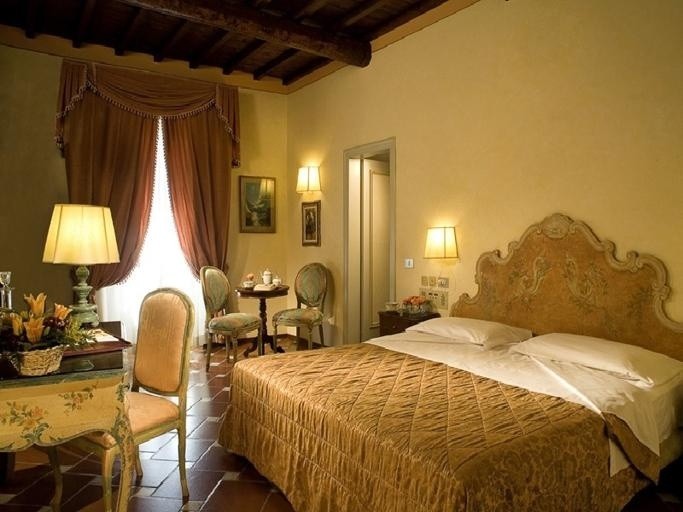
[46,287,196,511]
[272,262,329,351]
[200,265,263,372]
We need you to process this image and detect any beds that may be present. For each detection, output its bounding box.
[219,211,682,511]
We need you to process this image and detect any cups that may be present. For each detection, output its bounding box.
[272,276,281,287]
[261,267,272,284]
[384,302,400,311]
[243,281,253,288]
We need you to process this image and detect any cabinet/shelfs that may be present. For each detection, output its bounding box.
[378,310,441,336]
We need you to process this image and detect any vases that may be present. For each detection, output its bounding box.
[408,306,420,312]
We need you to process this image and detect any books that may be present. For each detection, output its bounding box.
[66,329,119,348]
[253,284,276,292]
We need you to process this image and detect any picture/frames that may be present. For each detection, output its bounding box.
[239,175,276,233]
[302,200,321,247]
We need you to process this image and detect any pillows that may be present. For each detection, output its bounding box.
[508,332,682,389]
[405,318,532,348]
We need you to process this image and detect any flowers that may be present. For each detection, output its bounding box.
[1,292,100,349]
[403,296,433,305]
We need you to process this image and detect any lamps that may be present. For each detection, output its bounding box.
[295,166,321,194]
[42,203,120,327]
[423,227,458,258]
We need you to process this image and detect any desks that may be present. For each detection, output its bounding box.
[0,326,136,485]
[0,356,135,511]
[237,284,289,357]
